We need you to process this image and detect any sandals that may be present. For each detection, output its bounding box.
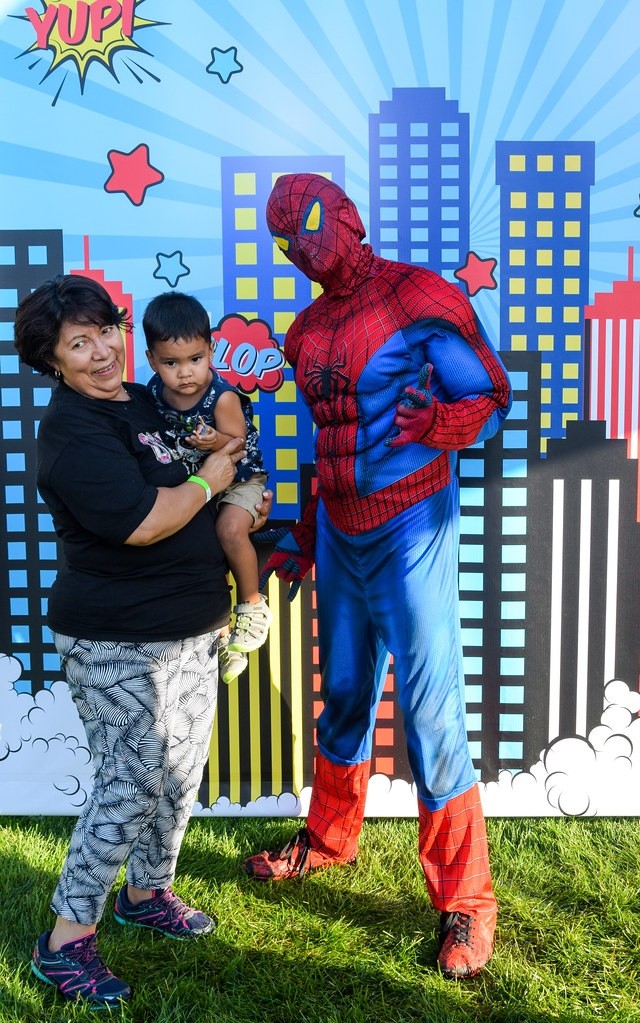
[228,593,273,652]
[218,634,248,684]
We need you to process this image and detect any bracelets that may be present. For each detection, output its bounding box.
[188,476,212,502]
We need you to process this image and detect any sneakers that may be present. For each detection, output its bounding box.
[114,883,215,941]
[242,828,338,882]
[439,905,497,979]
[31,930,131,1010]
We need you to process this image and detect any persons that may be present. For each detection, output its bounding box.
[14,273,271,1004]
[144,292,272,684]
[248,174,514,977]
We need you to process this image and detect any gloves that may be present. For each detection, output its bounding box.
[384,363,438,451]
[258,531,311,602]
